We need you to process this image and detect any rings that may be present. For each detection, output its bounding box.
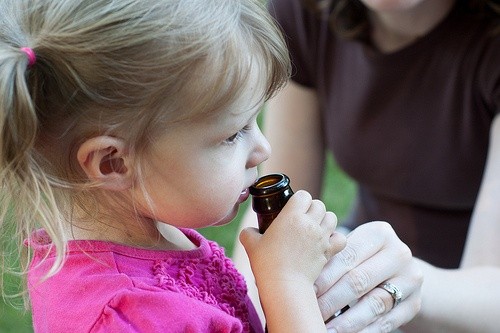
[377,281,403,309]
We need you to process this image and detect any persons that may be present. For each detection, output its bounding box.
[233,0,500,333]
[0,0,347,333]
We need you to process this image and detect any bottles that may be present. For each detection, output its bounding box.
[247,173,350,333]
